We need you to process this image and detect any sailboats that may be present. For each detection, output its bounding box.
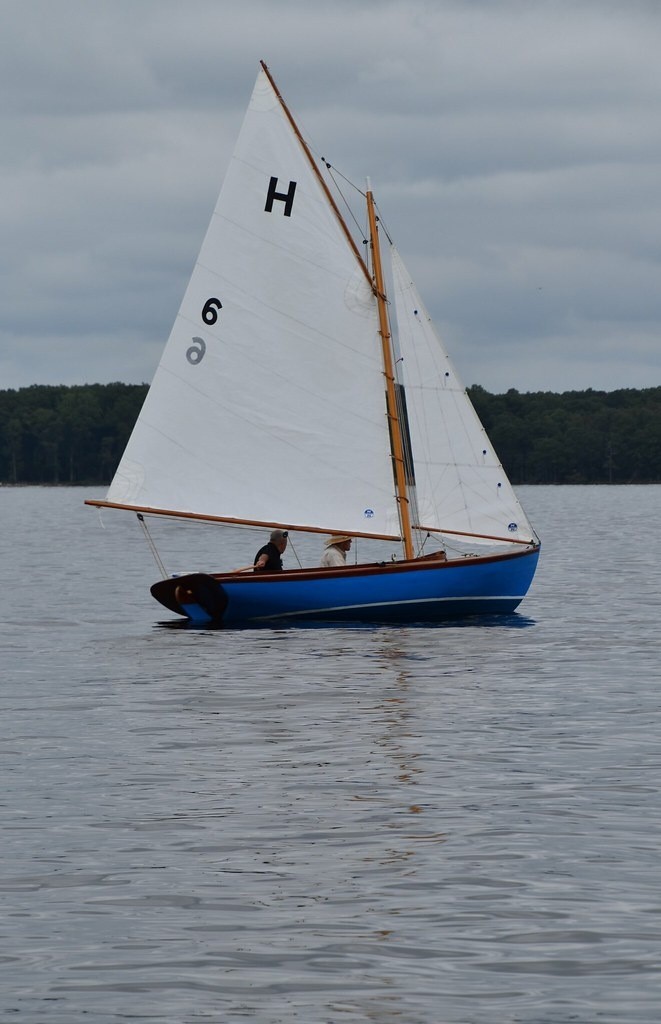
[85,60,541,632]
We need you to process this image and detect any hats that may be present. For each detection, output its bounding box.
[324,534,352,545]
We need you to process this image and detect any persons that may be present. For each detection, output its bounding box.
[254,530,287,572]
[319,534,353,567]
